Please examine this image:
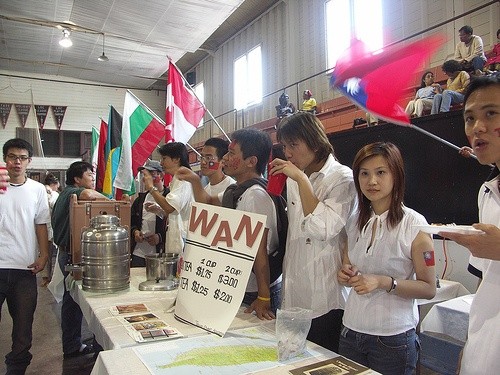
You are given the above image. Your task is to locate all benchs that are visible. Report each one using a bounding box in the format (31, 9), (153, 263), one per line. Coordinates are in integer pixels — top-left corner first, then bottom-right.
(188, 65), (463, 164)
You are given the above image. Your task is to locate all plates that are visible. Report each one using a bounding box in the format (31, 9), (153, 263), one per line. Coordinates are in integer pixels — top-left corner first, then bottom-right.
(411, 225), (485, 235)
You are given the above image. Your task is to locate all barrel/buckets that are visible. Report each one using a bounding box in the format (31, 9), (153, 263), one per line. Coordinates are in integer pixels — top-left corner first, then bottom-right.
(81, 215), (131, 293)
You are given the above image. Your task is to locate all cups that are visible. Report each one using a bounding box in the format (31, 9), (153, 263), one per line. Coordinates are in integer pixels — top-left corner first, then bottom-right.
(267, 163), (288, 196)
(0, 162), (7, 191)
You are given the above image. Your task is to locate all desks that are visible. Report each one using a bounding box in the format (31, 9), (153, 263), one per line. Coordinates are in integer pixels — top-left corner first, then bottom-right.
(416, 279), (472, 375)
(91, 323), (383, 375)
(420, 294), (475, 342)
(47, 248), (276, 349)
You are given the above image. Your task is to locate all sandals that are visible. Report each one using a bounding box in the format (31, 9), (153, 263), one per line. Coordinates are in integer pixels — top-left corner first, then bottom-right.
(64, 344), (94, 357)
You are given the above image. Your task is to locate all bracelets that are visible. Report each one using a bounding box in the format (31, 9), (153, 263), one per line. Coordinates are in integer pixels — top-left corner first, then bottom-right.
(257, 296), (270, 301)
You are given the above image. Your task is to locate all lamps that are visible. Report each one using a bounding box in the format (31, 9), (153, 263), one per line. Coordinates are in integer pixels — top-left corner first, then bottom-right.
(59, 27), (73, 47)
(98, 32), (109, 62)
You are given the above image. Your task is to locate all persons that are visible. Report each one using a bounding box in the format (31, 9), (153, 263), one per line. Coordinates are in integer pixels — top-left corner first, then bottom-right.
(0, 168), (10, 194)
(275, 92), (296, 124)
(336, 141), (437, 375)
(0, 26), (500, 375)
(268, 112), (355, 357)
(142, 142), (194, 261)
(51, 161), (111, 356)
(0, 138), (51, 375)
(201, 137), (237, 195)
(131, 158), (170, 267)
(224, 129), (279, 320)
(296, 90), (316, 115)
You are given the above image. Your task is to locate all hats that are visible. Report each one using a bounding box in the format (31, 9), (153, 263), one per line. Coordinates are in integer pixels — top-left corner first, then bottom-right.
(137, 160), (163, 172)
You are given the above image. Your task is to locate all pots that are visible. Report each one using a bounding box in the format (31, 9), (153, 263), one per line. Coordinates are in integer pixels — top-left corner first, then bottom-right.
(144, 252), (179, 281)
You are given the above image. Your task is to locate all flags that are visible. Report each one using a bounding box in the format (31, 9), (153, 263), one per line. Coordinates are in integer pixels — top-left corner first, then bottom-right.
(97, 120), (107, 194)
(162, 62), (207, 144)
(112, 92), (167, 192)
(91, 127), (100, 189)
(103, 108), (135, 200)
(330, 33), (445, 125)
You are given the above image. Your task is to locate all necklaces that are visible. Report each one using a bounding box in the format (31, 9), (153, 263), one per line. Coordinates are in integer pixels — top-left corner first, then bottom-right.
(10, 176), (27, 187)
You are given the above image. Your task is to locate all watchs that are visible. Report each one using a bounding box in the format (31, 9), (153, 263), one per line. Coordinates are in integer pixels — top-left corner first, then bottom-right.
(386, 277), (397, 293)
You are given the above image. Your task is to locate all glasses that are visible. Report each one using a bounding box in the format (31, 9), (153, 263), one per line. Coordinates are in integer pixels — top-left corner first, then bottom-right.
(5, 153), (30, 161)
(197, 156), (222, 161)
